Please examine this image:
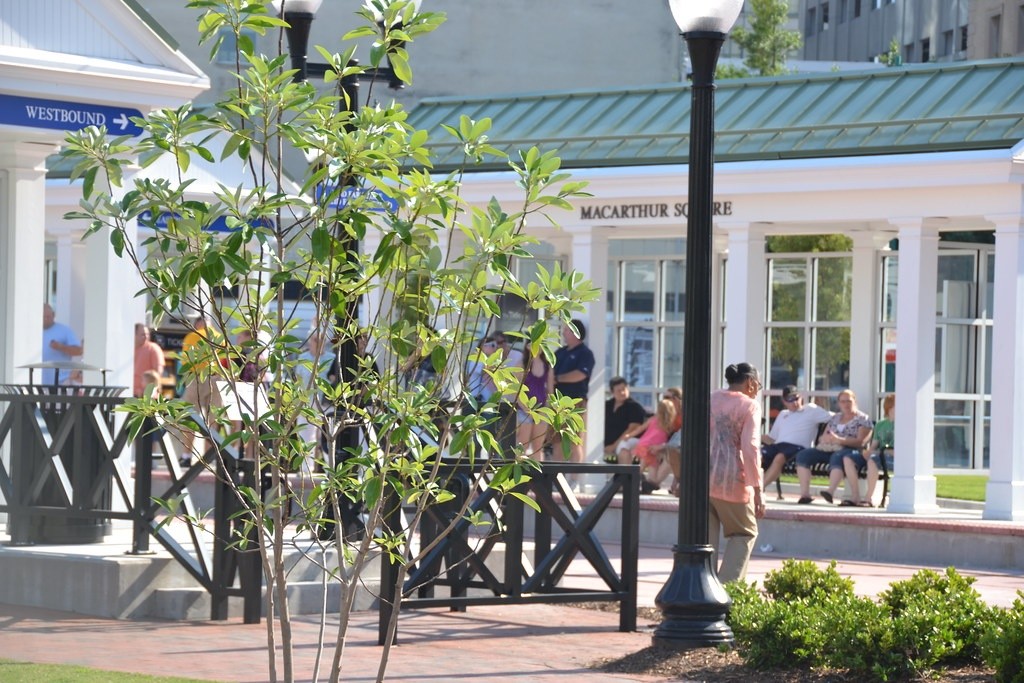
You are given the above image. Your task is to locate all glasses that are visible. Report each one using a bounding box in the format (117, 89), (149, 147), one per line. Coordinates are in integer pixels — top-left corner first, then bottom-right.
(750, 375), (763, 390)
(784, 396), (800, 402)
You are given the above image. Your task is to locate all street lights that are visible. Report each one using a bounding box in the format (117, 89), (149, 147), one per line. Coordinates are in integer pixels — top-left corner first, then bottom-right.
(650, 0), (744, 660)
(278, 2), (425, 547)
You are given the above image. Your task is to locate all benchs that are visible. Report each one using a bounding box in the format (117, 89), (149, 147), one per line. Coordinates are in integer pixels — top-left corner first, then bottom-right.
(766, 415), (895, 508)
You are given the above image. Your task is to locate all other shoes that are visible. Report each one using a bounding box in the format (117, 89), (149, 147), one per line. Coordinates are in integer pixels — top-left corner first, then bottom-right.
(798, 497), (814, 504)
(820, 491), (834, 503)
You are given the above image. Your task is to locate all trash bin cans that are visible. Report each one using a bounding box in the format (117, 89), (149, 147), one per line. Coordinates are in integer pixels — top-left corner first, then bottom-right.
(2, 356), (131, 539)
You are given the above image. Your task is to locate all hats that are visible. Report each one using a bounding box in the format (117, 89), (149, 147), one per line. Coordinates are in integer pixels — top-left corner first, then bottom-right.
(782, 384), (798, 398)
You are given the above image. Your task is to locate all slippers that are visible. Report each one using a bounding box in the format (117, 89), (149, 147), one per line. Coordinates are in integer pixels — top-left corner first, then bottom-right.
(857, 501), (874, 507)
(838, 500), (857, 507)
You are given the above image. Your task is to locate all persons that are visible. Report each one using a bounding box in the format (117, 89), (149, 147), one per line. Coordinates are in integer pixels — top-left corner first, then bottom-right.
(707, 362), (768, 585)
(760, 384), (841, 495)
(839, 395), (895, 507)
(173, 287), (680, 544)
(41, 303), (85, 385)
(134, 324), (166, 467)
(797, 390), (875, 503)
